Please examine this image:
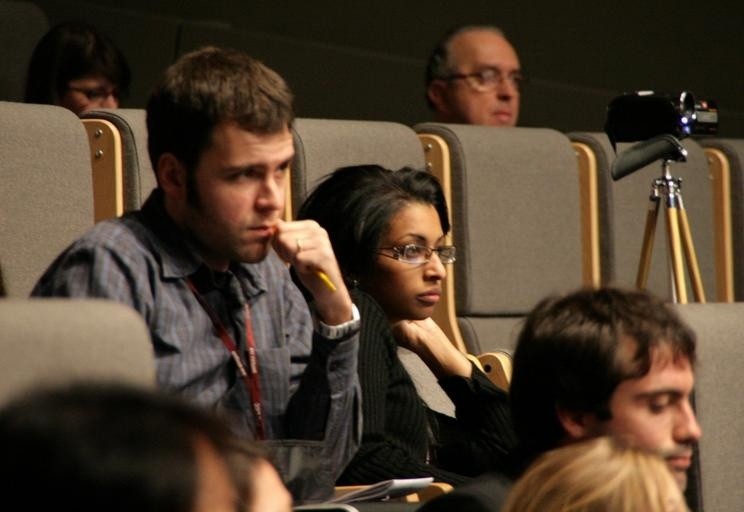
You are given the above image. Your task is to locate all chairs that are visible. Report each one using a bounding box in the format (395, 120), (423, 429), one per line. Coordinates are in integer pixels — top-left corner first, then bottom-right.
(2, 92), (95, 308)
(650, 306), (740, 507)
(275, 112), (450, 334)
(423, 114), (581, 308)
(97, 98), (262, 298)
(581, 118), (735, 303)
(0, 287), (164, 510)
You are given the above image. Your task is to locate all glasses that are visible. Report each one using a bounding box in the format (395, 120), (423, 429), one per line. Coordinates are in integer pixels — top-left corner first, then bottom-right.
(451, 70), (523, 89)
(372, 245), (457, 268)
(67, 83), (131, 100)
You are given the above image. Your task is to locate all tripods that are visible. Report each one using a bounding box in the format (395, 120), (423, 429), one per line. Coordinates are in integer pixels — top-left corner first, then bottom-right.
(635, 176), (707, 304)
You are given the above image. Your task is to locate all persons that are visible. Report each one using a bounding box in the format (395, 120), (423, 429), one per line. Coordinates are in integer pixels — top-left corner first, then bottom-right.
(29, 24), (121, 113)
(290, 163), (512, 493)
(415, 285), (704, 512)
(498, 434), (689, 512)
(218, 424), (295, 512)
(0, 379), (242, 512)
(26, 44), (365, 510)
(423, 22), (526, 127)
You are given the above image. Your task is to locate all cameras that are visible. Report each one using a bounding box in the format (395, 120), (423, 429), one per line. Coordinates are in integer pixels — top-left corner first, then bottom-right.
(603, 91), (720, 146)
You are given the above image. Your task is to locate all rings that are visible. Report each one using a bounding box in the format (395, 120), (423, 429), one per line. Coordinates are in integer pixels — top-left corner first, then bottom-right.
(295, 240), (305, 252)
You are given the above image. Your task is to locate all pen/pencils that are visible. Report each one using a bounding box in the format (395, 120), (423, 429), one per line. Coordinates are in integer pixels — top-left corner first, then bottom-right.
(315, 270), (336, 292)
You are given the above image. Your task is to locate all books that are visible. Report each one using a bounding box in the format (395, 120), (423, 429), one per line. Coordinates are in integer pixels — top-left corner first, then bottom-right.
(321, 476), (435, 504)
